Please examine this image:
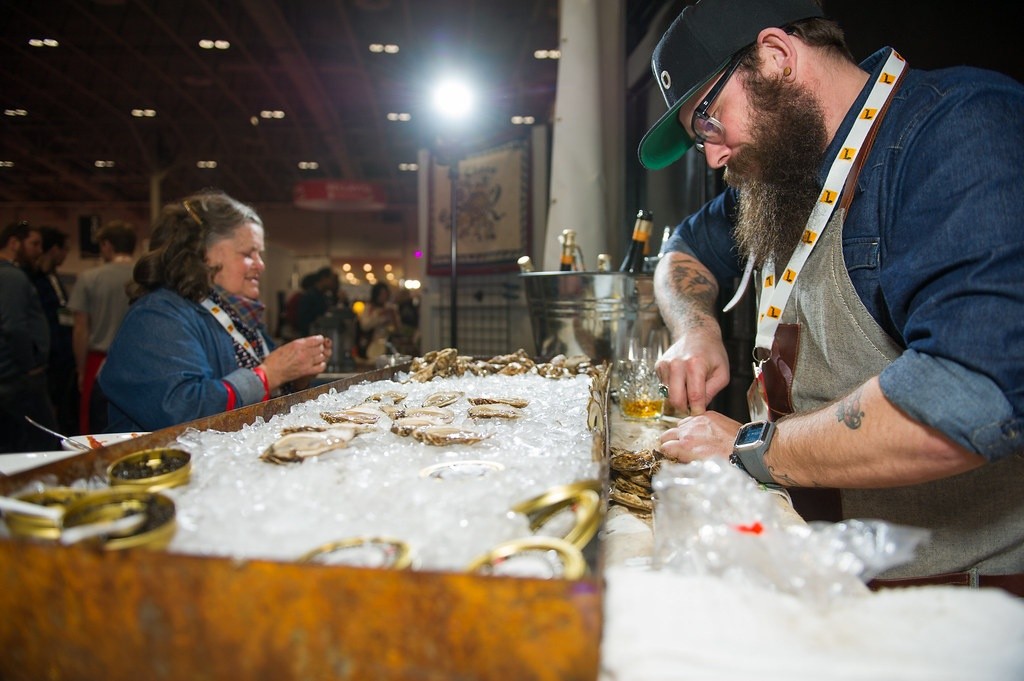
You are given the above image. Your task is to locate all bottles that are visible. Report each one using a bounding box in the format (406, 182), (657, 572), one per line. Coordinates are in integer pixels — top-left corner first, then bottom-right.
(597, 254), (612, 271)
(619, 209), (653, 273)
(517, 255), (536, 273)
(559, 230), (586, 271)
(660, 226), (674, 249)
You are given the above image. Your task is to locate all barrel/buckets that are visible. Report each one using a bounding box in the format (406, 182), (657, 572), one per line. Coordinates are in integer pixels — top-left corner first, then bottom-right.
(519, 271), (671, 359)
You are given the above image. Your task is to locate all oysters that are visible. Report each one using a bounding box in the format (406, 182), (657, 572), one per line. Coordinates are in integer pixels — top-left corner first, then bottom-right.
(254, 347), (612, 468)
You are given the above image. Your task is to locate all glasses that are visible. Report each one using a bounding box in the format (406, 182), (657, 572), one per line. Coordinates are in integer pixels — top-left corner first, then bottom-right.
(17, 220), (28, 226)
(691, 27), (795, 155)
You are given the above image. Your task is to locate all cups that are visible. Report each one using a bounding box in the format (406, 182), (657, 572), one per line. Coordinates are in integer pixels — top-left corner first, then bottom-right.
(622, 347), (666, 421)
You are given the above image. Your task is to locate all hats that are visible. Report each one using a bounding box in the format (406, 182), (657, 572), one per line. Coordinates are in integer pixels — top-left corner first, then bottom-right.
(637, 0), (825, 171)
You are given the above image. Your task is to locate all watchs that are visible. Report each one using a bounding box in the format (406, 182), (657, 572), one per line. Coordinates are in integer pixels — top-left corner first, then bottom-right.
(732, 420), (785, 490)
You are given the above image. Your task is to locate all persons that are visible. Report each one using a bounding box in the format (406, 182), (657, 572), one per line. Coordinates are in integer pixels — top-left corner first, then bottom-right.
(0, 219), (139, 454)
(638, 0), (1023, 606)
(276, 267), (420, 372)
(86, 191), (335, 439)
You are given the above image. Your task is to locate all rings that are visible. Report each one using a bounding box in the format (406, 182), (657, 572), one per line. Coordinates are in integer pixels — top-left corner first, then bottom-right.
(657, 383), (670, 398)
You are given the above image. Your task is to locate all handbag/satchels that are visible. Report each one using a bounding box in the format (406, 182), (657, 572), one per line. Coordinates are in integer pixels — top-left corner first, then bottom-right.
(354, 322), (375, 358)
